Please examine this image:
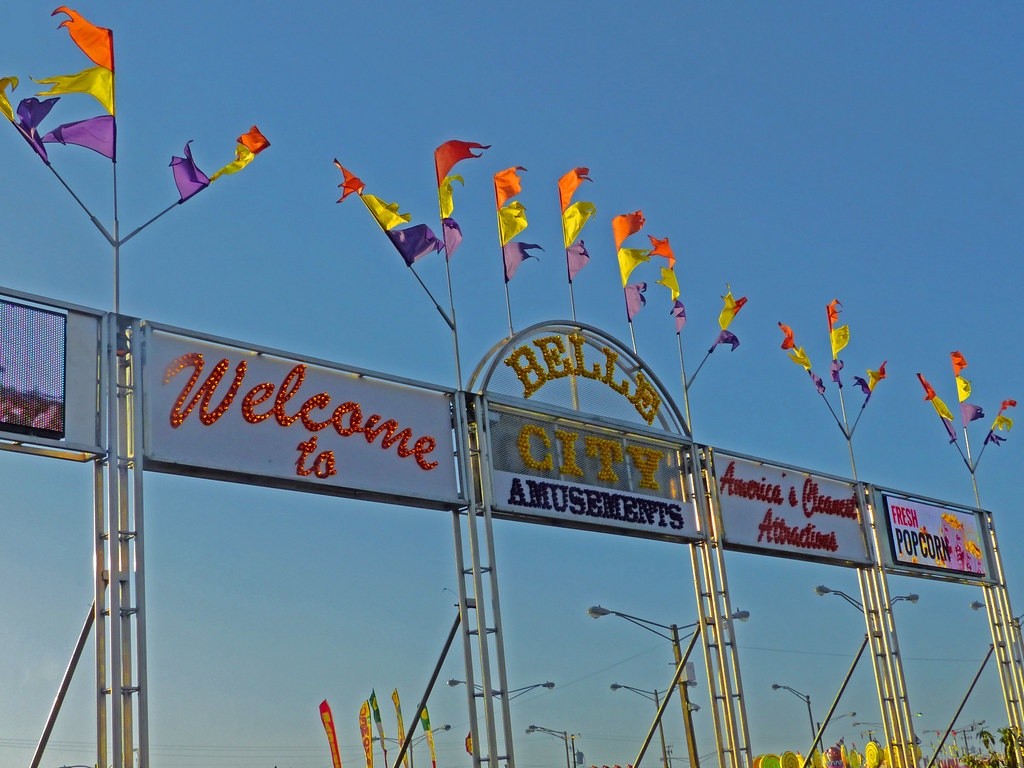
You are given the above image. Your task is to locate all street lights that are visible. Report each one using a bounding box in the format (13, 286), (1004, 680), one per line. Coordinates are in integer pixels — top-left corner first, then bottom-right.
(587, 604), (750, 768)
(962, 721), (987, 754)
(526, 724), (570, 768)
(370, 725), (453, 768)
(816, 712), (858, 754)
(922, 728), (960, 758)
(609, 680), (700, 768)
(772, 683), (816, 743)
(970, 600), (1024, 668)
